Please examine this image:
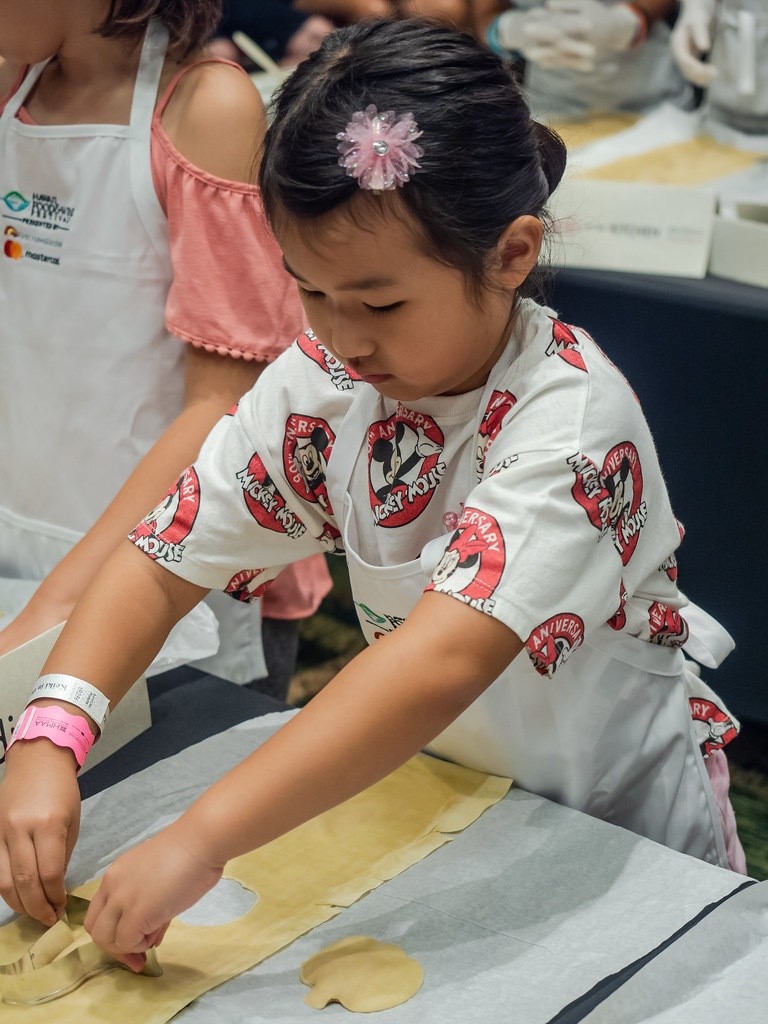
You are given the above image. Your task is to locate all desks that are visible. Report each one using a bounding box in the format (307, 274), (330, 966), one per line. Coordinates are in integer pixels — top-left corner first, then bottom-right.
(525, 97), (768, 769)
(1, 662), (768, 1024)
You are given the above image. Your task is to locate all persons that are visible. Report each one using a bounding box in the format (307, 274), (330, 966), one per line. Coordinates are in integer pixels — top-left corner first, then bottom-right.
(199, 0), (670, 68)
(0, 1), (335, 697)
(0, 1), (750, 976)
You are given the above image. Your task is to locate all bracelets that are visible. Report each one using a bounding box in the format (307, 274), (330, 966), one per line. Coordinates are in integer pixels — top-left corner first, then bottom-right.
(3, 671), (113, 776)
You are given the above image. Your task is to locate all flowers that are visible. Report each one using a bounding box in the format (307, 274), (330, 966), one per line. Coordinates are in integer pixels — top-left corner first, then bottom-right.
(335, 104), (425, 196)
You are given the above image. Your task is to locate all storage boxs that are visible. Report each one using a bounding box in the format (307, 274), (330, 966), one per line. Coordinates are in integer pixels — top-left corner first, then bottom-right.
(1, 574), (151, 780)
